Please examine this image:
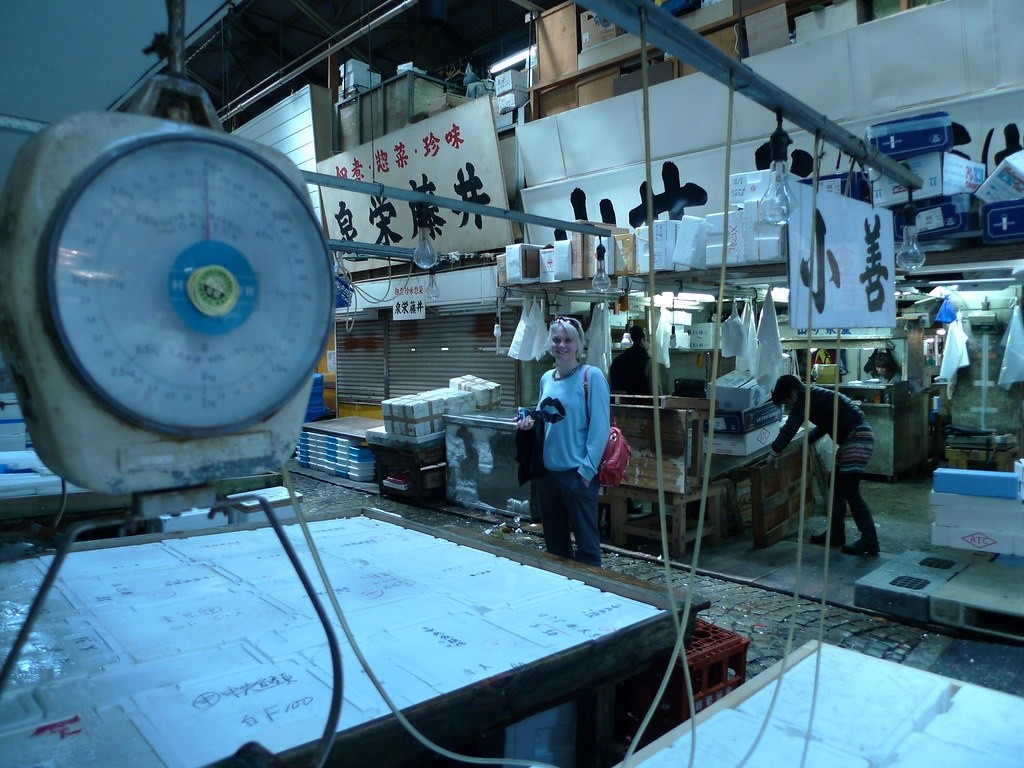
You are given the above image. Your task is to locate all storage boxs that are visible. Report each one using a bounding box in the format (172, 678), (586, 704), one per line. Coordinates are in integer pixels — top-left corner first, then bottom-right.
(928, 458), (1024, 568)
(292, 372), (502, 509)
(813, 363), (837, 383)
(671, 368), (795, 524)
(669, 616), (752, 727)
(339, 1), (1024, 290)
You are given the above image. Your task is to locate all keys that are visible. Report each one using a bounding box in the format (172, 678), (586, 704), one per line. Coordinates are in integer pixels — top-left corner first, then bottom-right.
(529, 418), (535, 422)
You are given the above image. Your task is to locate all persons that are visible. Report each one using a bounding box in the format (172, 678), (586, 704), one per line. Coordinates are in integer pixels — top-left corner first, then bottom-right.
(515, 316), (632, 567)
(767, 374), (881, 555)
(864, 355), (901, 382)
(609, 325), (663, 406)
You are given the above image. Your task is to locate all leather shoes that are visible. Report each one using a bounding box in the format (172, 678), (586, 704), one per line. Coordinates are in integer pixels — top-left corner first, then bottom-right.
(842, 539), (880, 553)
(810, 531), (845, 544)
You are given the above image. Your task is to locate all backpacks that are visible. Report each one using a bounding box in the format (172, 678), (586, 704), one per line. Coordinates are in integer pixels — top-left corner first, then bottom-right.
(597, 407), (632, 487)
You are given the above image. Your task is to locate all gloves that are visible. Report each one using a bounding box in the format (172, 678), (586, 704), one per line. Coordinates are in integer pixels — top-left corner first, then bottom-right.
(767, 454), (782, 469)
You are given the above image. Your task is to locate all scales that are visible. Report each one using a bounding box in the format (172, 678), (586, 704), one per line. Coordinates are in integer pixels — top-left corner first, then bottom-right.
(0, 0), (340, 768)
